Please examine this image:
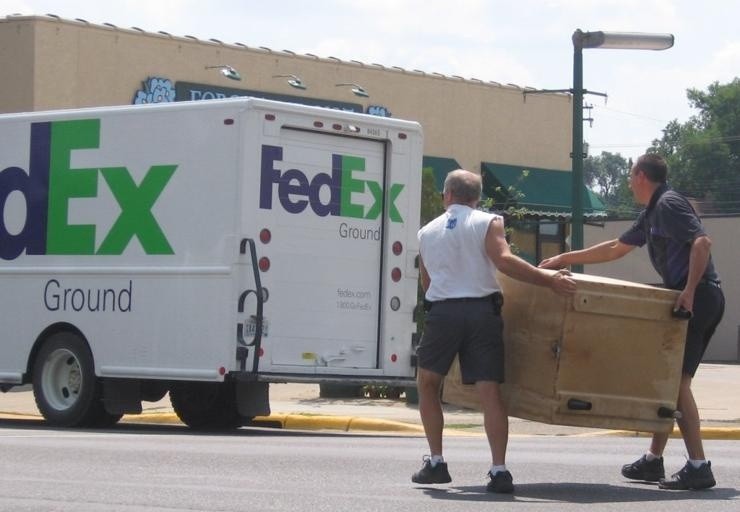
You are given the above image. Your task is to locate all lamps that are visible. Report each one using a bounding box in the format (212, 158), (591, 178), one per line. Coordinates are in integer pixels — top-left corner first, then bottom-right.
(205, 63), (371, 99)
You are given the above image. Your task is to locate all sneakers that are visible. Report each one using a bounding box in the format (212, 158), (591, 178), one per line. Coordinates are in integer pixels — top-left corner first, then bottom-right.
(486, 470), (514, 493)
(623, 455), (664, 481)
(658, 461), (716, 489)
(412, 459), (451, 484)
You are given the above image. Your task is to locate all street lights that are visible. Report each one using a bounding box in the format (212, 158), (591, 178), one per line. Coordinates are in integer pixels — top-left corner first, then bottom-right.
(569, 27), (675, 276)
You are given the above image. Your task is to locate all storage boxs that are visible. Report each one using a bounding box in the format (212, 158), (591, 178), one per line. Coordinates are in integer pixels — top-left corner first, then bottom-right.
(440, 266), (692, 434)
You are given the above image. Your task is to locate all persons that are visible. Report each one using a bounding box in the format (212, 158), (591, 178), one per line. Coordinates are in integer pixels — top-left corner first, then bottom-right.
(535, 152), (725, 490)
(411, 166), (579, 493)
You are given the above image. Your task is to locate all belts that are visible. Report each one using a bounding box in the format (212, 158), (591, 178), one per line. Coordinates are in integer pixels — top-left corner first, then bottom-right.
(702, 278), (720, 288)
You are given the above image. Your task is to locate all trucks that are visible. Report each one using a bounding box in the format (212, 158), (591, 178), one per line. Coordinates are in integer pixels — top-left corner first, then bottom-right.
(0, 95), (428, 434)
